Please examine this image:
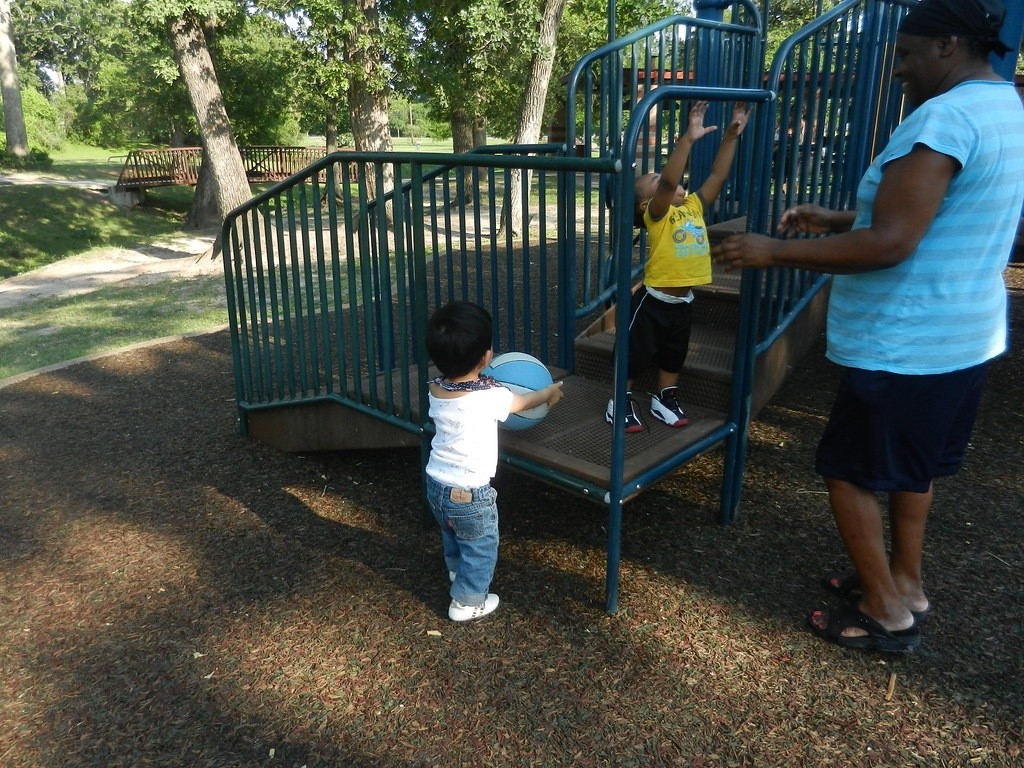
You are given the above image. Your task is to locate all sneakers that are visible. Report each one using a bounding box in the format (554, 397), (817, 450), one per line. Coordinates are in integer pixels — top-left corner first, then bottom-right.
(448, 592), (499, 622)
(650, 384), (689, 426)
(449, 571), (456, 583)
(605, 391), (644, 433)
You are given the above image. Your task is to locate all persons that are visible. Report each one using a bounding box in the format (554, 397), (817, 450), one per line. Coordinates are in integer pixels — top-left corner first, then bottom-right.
(426, 303), (564, 622)
(711, 0), (1024, 654)
(603, 99), (752, 433)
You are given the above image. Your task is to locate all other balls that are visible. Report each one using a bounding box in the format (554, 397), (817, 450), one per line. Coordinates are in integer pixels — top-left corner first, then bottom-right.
(482, 352), (554, 431)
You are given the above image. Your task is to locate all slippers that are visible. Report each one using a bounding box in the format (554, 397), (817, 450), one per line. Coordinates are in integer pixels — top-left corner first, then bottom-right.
(826, 574), (931, 624)
(807, 603), (922, 652)
